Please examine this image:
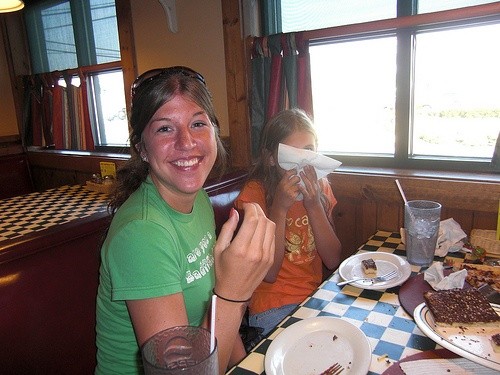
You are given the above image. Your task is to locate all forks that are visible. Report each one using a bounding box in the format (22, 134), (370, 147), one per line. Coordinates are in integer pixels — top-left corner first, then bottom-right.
(336, 270), (399, 286)
(320, 362), (344, 375)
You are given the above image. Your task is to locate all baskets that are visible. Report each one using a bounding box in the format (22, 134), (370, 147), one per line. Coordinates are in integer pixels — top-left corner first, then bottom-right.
(470, 229), (500, 254)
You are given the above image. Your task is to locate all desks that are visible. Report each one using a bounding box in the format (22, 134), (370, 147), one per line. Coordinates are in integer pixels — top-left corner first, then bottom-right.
(0, 182), (122, 243)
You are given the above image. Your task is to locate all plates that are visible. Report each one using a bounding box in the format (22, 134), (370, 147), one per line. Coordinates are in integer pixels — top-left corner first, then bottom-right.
(338, 252), (412, 290)
(413, 302), (500, 372)
(265, 316), (372, 375)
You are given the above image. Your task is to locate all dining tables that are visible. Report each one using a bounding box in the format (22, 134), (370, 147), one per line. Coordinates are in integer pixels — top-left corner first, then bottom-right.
(226, 230), (500, 375)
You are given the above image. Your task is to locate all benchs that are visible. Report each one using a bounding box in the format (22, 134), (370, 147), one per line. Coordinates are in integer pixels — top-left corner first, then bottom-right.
(0, 153), (32, 200)
(0, 165), (259, 375)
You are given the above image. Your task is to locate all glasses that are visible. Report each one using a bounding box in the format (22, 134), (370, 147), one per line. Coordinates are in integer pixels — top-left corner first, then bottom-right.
(130, 66), (205, 105)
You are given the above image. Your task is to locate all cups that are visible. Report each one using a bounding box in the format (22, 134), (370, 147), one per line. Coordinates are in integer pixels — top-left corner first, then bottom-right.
(404, 200), (442, 266)
(142, 325), (219, 375)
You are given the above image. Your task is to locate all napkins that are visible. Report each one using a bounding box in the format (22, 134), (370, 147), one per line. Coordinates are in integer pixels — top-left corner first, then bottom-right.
(400, 217), (467, 257)
(278, 143), (342, 187)
(424, 261), (467, 291)
(399, 358), (500, 375)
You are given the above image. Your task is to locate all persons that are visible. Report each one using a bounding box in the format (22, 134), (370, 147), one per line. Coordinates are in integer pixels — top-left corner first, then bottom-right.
(236, 110), (342, 338)
(95, 68), (276, 375)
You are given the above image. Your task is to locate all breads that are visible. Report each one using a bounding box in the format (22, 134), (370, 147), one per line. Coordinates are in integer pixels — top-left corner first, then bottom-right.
(362, 259), (377, 274)
(452, 262), (500, 292)
(424, 289), (500, 350)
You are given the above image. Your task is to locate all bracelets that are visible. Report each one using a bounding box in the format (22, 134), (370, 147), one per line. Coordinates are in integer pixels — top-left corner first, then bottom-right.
(212, 288), (251, 302)
